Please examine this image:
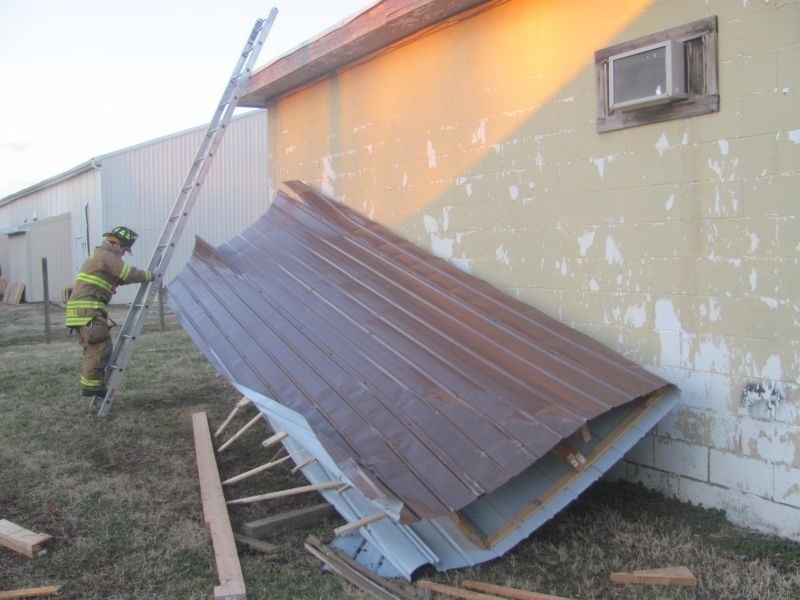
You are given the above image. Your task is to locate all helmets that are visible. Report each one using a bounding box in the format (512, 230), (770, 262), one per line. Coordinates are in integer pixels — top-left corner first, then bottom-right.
(102, 227), (139, 246)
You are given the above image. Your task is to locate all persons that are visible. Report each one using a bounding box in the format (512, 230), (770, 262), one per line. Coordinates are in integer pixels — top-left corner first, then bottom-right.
(68, 226), (155, 398)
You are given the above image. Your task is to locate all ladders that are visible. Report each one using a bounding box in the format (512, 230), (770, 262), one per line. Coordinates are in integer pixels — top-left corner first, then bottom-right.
(90, 6), (279, 418)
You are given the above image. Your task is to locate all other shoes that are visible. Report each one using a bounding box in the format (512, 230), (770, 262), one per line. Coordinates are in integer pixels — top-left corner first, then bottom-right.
(82, 387), (114, 403)
